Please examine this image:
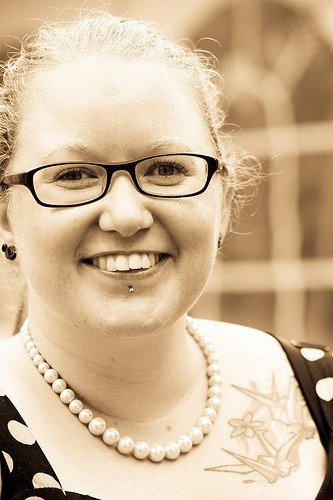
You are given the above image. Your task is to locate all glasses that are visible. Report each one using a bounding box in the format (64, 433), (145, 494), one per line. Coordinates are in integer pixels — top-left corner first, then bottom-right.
(1, 153), (224, 207)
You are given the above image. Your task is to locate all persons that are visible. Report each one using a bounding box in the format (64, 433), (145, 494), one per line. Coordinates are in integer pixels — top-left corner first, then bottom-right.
(0, 13), (332, 500)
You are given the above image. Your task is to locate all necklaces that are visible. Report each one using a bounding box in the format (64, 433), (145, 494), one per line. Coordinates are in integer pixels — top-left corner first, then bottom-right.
(19, 315), (224, 463)
